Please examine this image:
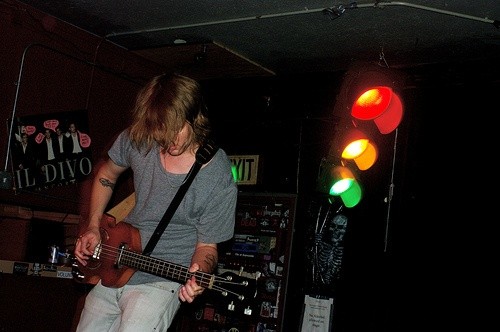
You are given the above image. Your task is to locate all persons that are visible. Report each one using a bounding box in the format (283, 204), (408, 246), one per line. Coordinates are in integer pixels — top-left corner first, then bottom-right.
(58, 69), (243, 332)
(9, 117), (84, 170)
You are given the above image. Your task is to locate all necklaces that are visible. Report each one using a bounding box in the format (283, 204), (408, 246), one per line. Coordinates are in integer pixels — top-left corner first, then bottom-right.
(161, 152), (200, 188)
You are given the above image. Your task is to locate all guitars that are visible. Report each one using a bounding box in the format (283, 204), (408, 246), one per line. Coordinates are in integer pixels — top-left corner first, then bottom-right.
(72, 212), (261, 315)
